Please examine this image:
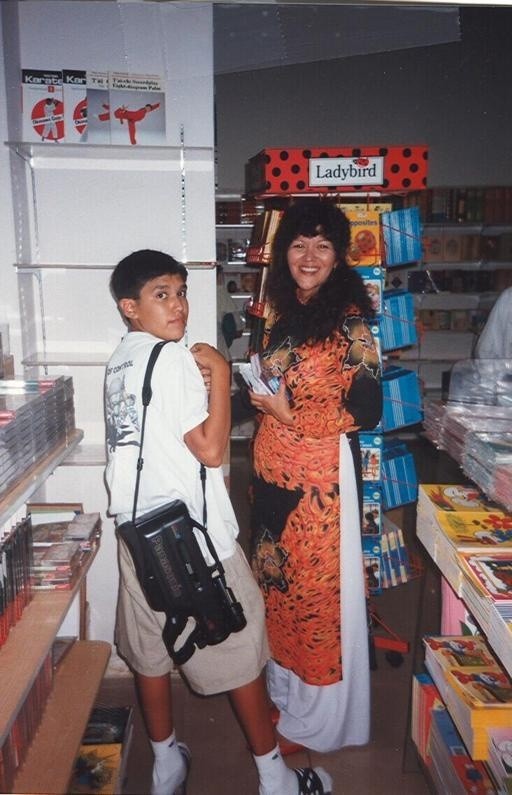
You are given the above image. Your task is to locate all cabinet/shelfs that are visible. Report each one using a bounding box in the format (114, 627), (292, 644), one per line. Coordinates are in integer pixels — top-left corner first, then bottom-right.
(3, 125), (215, 477)
(216, 180), (510, 441)
(0, 416), (142, 794)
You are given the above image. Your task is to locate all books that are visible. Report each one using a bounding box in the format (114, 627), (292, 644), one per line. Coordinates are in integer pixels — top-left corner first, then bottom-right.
(0, 372), (102, 794)
(403, 187), (512, 332)
(240, 351), (292, 413)
(81, 703), (132, 742)
(409, 390), (512, 794)
(68, 743), (123, 795)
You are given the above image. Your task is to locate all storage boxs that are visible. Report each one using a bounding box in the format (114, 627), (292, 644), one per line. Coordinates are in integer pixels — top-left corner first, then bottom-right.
(243, 142), (432, 195)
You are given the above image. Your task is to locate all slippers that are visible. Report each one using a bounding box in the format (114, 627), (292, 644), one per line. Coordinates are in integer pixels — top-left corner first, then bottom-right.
(258, 766), (333, 795)
(150, 742), (193, 795)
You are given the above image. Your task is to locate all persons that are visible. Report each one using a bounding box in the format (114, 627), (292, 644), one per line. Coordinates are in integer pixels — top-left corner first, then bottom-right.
(100, 244), (333, 795)
(357, 448), (383, 594)
(232, 198), (383, 760)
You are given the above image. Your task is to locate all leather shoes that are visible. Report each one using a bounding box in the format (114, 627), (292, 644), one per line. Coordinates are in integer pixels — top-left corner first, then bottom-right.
(277, 734), (304, 755)
(268, 703), (281, 725)
